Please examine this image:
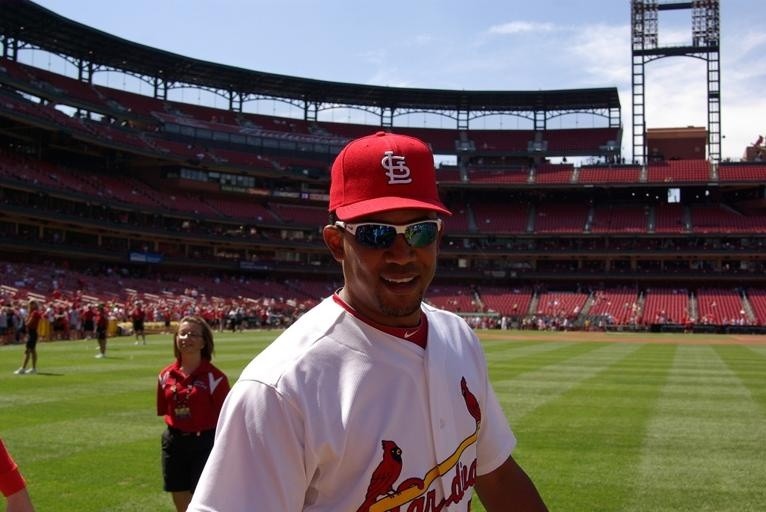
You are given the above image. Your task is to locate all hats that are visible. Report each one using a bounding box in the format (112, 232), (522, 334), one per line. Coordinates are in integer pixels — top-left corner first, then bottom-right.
(329, 131), (452, 220)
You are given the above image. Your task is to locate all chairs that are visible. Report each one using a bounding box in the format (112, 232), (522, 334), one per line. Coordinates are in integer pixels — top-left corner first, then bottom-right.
(0, 55), (766, 339)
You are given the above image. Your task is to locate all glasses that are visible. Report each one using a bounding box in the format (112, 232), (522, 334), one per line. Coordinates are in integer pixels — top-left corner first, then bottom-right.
(335, 219), (441, 250)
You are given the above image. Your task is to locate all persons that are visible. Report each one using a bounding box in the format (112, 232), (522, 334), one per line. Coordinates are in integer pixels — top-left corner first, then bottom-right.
(182, 130), (554, 512)
(0, 439), (33, 512)
(2, 237), (760, 341)
(91, 304), (109, 359)
(155, 314), (231, 512)
(13, 300), (40, 375)
(129, 302), (147, 345)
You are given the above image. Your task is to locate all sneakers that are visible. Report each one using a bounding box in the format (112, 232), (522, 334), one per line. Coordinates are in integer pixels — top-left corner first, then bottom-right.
(96, 353), (107, 359)
(24, 368), (37, 374)
(14, 367), (24, 374)
